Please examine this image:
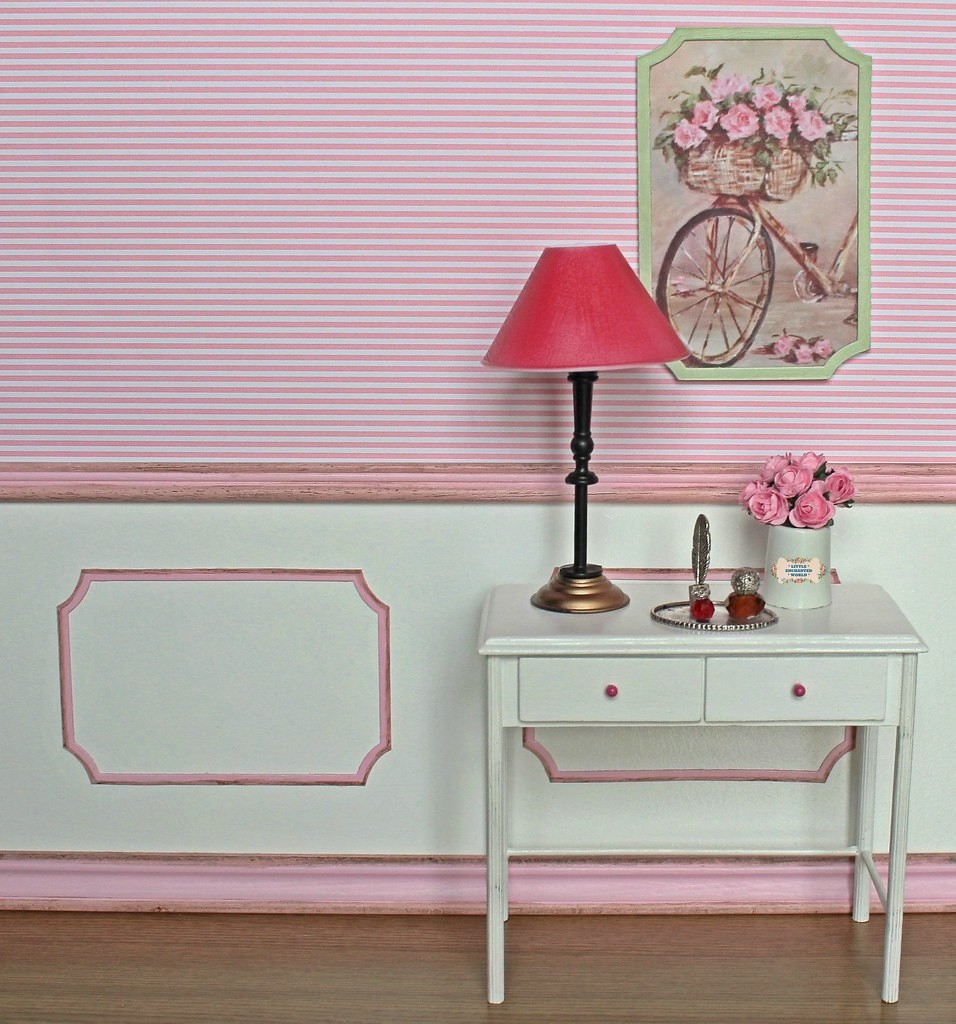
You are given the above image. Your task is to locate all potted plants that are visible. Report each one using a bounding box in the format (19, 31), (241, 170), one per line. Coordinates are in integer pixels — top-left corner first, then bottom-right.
(740, 451), (857, 617)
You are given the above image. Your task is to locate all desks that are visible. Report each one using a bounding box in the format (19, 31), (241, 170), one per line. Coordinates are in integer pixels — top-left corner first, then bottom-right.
(474, 575), (932, 1008)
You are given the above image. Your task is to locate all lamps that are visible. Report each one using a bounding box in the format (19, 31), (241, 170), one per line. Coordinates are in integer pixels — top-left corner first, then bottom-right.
(480, 241), (692, 618)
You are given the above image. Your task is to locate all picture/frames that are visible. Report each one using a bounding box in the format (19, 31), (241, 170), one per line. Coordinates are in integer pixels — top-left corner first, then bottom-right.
(633, 22), (877, 385)
